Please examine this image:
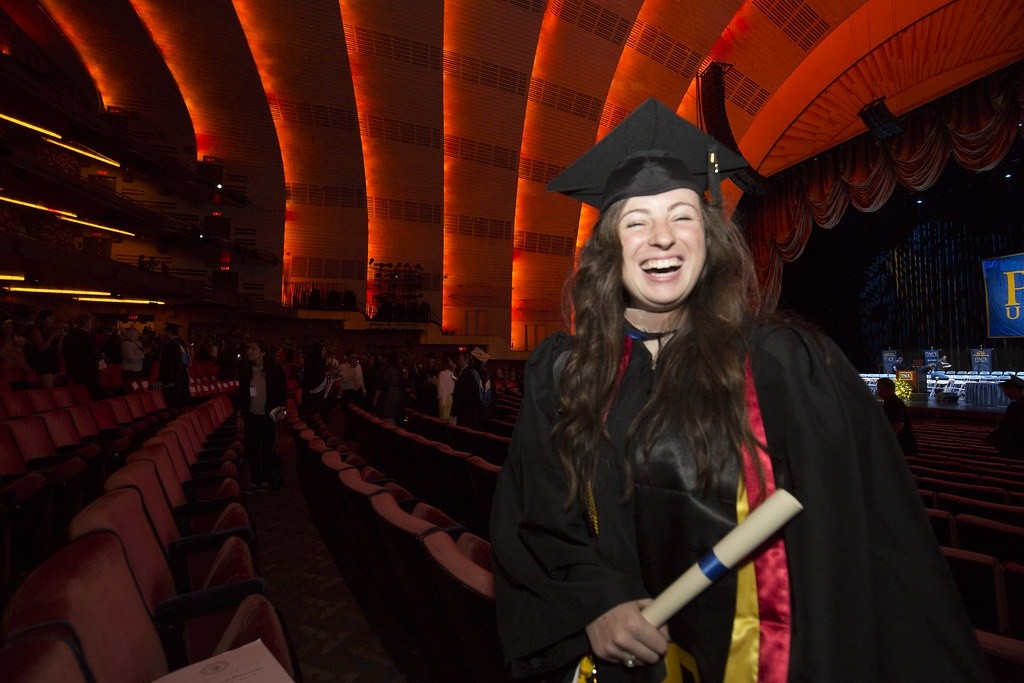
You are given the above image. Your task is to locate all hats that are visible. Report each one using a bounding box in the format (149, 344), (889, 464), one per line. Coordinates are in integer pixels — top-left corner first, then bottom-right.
(546, 98), (747, 224)
(164, 322), (181, 330)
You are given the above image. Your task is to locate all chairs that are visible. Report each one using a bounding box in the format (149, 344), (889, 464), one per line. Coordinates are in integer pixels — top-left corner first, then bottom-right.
(0, 316), (1024, 683)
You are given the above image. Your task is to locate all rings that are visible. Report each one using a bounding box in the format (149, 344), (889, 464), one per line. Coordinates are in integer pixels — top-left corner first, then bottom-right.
(624, 655), (635, 667)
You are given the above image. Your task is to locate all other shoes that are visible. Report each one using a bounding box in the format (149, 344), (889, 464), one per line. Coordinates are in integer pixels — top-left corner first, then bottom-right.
(244, 483), (257, 495)
(258, 481), (270, 492)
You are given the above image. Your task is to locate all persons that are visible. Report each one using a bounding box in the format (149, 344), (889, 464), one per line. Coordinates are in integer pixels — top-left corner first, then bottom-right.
(490, 98), (997, 682)
(0, 304), (193, 408)
(198, 334), (282, 362)
(286, 345), (296, 361)
(236, 340), (289, 494)
(367, 346), (492, 426)
(391, 283), (431, 323)
(300, 342), (366, 410)
(984, 375), (1024, 459)
(877, 379), (919, 456)
